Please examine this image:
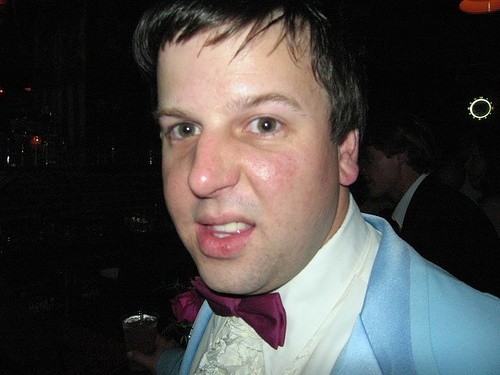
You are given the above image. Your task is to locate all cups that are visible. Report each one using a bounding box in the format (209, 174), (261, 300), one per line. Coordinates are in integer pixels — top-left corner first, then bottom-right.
(122, 314), (159, 371)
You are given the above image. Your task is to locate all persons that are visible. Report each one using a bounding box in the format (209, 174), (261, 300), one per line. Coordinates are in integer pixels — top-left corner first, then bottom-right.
(122, 0), (500, 375)
(358, 112), (500, 298)
(427, 113), (500, 232)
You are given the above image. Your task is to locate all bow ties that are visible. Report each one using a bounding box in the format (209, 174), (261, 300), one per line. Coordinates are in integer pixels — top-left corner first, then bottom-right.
(191, 276), (286, 351)
